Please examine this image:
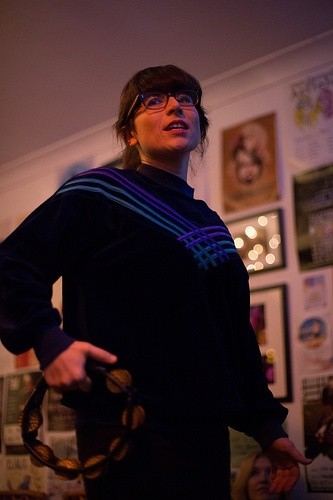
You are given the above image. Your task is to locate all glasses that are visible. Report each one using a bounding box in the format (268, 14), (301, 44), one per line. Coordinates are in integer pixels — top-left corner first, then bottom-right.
(124, 91), (199, 123)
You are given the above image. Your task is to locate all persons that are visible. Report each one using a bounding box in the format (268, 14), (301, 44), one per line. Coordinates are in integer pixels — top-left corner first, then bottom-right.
(231, 450), (284, 500)
(1, 62), (313, 500)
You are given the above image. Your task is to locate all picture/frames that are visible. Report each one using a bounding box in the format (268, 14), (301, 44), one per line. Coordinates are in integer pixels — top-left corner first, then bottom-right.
(249, 282), (294, 403)
(223, 207), (287, 275)
(219, 110), (282, 216)
